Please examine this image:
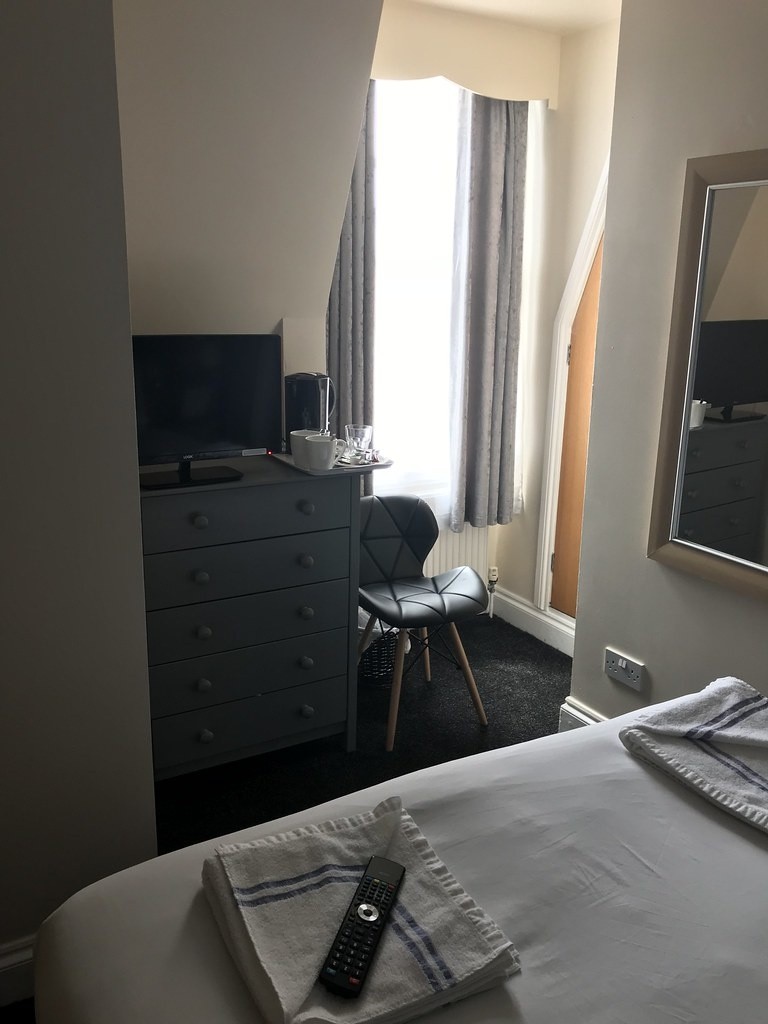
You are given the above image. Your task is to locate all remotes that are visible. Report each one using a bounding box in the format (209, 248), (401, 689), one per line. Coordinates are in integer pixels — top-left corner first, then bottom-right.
(318, 855), (406, 1000)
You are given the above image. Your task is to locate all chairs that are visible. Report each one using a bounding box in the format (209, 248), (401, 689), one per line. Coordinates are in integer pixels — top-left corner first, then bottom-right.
(353, 495), (488, 751)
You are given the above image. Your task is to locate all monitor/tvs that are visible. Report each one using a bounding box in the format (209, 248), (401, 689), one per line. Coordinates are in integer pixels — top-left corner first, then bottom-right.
(693, 319), (768, 423)
(133, 334), (283, 491)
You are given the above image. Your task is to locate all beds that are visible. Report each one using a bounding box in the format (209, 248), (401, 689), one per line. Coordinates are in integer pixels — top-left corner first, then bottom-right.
(23, 674), (768, 1024)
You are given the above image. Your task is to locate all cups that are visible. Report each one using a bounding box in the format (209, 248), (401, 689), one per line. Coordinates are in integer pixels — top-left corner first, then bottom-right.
(345, 424), (373, 459)
(304, 429), (330, 436)
(290, 430), (324, 466)
(305, 436), (346, 471)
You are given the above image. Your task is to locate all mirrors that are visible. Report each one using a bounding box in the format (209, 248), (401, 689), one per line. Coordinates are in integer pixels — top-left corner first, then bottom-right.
(644, 148), (768, 602)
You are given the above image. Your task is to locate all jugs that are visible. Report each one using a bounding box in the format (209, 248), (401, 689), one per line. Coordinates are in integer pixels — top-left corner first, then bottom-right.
(285, 372), (336, 455)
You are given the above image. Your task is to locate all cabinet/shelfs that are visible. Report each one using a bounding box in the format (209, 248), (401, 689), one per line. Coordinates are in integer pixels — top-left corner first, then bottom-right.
(678, 416), (768, 562)
(140, 454), (372, 781)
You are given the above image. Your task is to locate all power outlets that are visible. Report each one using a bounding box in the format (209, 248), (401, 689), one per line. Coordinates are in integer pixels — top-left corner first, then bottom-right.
(603, 644), (646, 693)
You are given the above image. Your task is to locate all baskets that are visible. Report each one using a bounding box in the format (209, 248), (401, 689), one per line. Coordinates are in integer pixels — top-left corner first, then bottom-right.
(359, 628), (398, 687)
